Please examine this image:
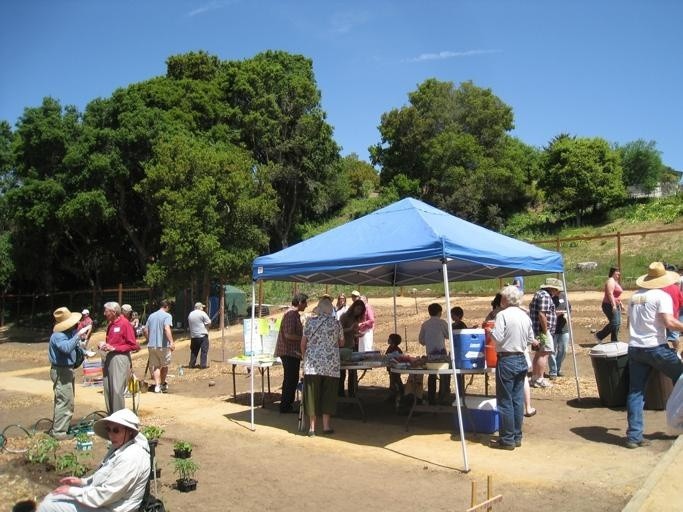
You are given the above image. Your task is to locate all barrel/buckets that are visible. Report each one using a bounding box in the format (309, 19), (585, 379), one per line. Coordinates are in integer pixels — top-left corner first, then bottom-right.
(482, 320), (498, 367)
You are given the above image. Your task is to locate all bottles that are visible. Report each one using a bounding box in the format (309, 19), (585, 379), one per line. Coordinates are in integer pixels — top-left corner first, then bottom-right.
(177, 364), (183, 376)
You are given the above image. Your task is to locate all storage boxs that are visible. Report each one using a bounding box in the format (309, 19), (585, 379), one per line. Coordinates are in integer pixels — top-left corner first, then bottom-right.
(452, 395), (500, 434)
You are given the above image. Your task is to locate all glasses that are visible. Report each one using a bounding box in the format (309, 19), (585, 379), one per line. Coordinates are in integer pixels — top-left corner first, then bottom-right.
(105, 427), (119, 433)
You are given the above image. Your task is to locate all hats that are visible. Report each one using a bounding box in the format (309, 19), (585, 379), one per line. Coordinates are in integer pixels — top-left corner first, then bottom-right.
(539, 278), (564, 292)
(635, 261), (679, 289)
(194, 302), (205, 307)
(350, 290), (359, 296)
(93, 408), (139, 440)
(52, 307), (82, 332)
(318, 294), (333, 302)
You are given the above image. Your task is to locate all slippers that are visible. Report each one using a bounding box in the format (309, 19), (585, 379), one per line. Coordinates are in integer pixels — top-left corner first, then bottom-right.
(526, 408), (535, 417)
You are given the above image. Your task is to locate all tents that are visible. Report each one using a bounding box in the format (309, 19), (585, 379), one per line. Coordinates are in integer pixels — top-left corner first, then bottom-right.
(176, 284), (248, 329)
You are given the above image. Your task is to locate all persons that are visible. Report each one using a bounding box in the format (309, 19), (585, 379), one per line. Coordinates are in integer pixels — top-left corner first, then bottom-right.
(187, 302), (211, 369)
(272, 290), (374, 436)
(76, 302), (140, 416)
(385, 333), (404, 394)
(38, 408), (151, 512)
(143, 300), (175, 394)
(48, 306), (96, 436)
(486, 261), (683, 451)
(451, 307), (467, 398)
(419, 303), (449, 406)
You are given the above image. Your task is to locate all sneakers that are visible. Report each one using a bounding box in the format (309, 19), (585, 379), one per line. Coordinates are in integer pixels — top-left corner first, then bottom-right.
(53, 435), (73, 441)
(488, 439), (520, 450)
(626, 439), (651, 448)
(530, 372), (564, 388)
(154, 380), (167, 392)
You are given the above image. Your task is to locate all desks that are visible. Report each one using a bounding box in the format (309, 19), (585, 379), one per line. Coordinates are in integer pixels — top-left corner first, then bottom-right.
(337, 360), (387, 422)
(390, 362), (500, 434)
(227, 356), (299, 409)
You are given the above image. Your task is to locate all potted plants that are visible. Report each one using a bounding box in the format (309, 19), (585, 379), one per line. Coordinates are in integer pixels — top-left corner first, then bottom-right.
(173, 440), (193, 459)
(141, 425), (166, 457)
(150, 462), (161, 479)
(173, 459), (199, 491)
(74, 433), (94, 452)
